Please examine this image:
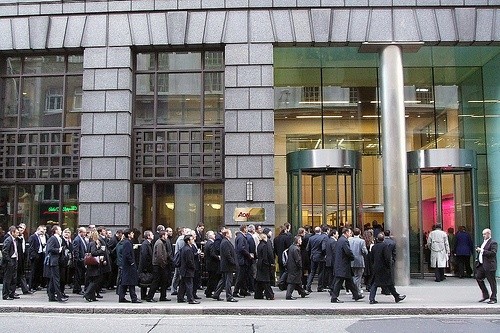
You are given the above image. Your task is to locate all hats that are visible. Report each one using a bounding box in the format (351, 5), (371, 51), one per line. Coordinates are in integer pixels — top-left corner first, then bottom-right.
(263, 228), (271, 234)
(436, 223), (442, 227)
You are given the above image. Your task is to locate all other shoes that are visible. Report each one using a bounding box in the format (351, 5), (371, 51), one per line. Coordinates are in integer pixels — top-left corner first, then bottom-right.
(435, 279), (440, 282)
(381, 291), (391, 295)
(395, 295), (406, 303)
(479, 298), (487, 302)
(368, 288), (371, 291)
(459, 275), (464, 278)
(370, 300), (378, 304)
(467, 274), (471, 278)
(488, 299), (496, 303)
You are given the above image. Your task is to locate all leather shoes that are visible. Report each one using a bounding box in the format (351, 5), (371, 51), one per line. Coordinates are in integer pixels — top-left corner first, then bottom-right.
(2, 285), (366, 304)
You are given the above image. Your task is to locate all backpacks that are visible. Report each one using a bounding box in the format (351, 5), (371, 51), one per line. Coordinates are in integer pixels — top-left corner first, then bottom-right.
(281, 248), (288, 266)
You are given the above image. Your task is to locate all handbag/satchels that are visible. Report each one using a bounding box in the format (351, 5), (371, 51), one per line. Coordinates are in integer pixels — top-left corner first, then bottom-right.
(85, 253), (100, 266)
(171, 248), (181, 268)
(139, 271), (153, 287)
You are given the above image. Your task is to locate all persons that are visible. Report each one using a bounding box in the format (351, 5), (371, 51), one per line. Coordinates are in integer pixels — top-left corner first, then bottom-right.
(426, 223), (450, 282)
(330, 228), (365, 303)
(171, 222), (205, 298)
(2, 223), (33, 300)
(456, 226), (473, 277)
(212, 229), (239, 302)
(369, 233), (406, 304)
(214, 225), (228, 292)
(274, 221), (352, 300)
(112, 228), (142, 303)
(177, 236), (200, 304)
(235, 223), (276, 299)
(72, 224), (111, 302)
(204, 231), (221, 298)
(348, 219), (396, 298)
(140, 224), (173, 302)
(410, 226), (428, 255)
(474, 228), (498, 303)
(447, 227), (458, 274)
(29, 224), (73, 302)
